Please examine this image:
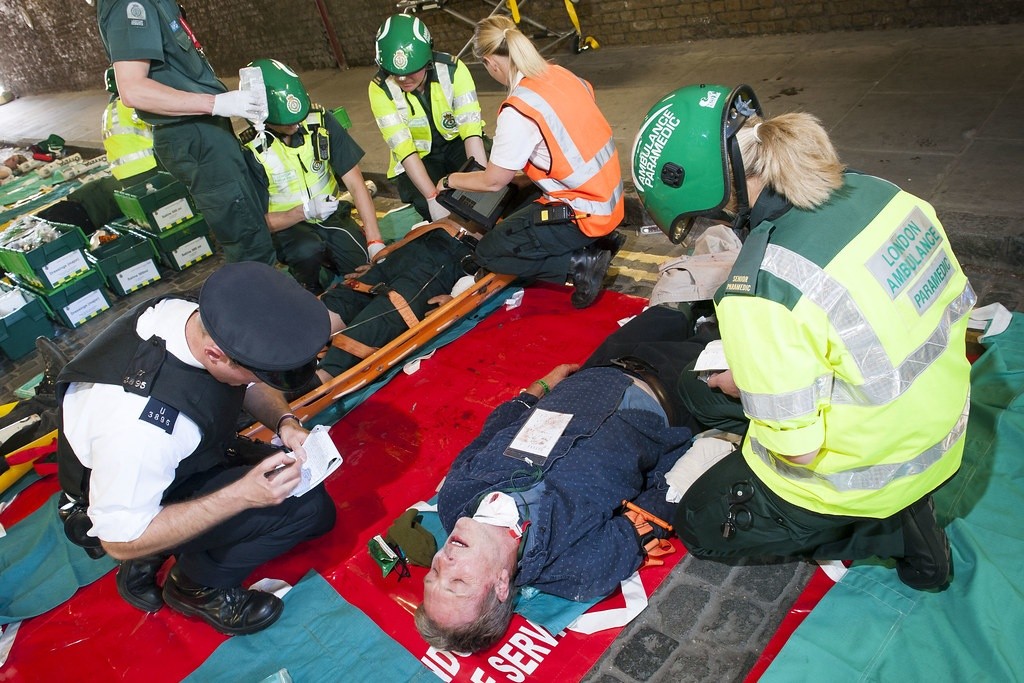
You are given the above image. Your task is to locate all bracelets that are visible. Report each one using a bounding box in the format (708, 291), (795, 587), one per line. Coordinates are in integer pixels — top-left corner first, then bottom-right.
(443, 173), (451, 188)
(276, 413), (303, 438)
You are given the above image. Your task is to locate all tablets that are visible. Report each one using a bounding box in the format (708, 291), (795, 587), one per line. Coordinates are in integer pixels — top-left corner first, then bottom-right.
(443, 162), (517, 230)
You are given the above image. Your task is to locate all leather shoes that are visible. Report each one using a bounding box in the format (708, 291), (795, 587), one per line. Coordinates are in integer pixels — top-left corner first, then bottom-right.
(594, 231), (627, 261)
(162, 573), (283, 636)
(35, 336), (71, 393)
(117, 557), (161, 613)
(571, 246), (611, 309)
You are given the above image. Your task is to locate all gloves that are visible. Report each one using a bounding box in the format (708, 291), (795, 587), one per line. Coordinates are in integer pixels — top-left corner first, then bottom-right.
(427, 195), (451, 221)
(212, 90), (263, 119)
(367, 242), (387, 264)
(304, 195), (339, 221)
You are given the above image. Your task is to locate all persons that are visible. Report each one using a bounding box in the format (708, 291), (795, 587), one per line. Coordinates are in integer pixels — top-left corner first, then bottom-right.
(630, 82), (977, 594)
(413, 293), (720, 655)
(100, 67), (160, 187)
(284, 213), (489, 403)
(97, 0), (273, 266)
(239, 58), (386, 295)
(55, 260), (336, 634)
(436, 15), (627, 308)
(368, 14), (490, 224)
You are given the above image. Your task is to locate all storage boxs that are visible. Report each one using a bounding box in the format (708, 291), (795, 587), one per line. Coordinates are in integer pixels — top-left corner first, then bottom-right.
(4, 258), (113, 332)
(0, 281), (55, 362)
(0, 214), (93, 292)
(83, 225), (162, 298)
(112, 212), (216, 273)
(112, 171), (198, 235)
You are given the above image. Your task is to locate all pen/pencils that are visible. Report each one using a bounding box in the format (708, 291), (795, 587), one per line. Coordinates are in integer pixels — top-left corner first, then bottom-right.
(326, 195), (329, 203)
(263, 463), (293, 478)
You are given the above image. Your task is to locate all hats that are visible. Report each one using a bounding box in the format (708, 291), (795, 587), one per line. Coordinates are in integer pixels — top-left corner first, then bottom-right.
(199, 261), (331, 392)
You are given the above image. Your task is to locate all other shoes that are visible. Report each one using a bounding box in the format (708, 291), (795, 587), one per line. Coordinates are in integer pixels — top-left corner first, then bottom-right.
(897, 496), (950, 591)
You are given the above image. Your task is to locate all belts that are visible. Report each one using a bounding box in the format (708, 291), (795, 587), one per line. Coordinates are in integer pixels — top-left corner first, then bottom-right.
(152, 116), (200, 132)
(612, 357), (681, 428)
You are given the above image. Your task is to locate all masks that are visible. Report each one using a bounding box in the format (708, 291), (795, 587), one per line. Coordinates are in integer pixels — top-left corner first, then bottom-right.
(472, 491), (520, 526)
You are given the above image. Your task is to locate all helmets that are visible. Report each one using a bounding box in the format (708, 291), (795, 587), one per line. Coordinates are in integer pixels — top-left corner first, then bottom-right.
(103, 67), (119, 93)
(246, 58), (311, 125)
(375, 14), (434, 75)
(630, 84), (763, 245)
